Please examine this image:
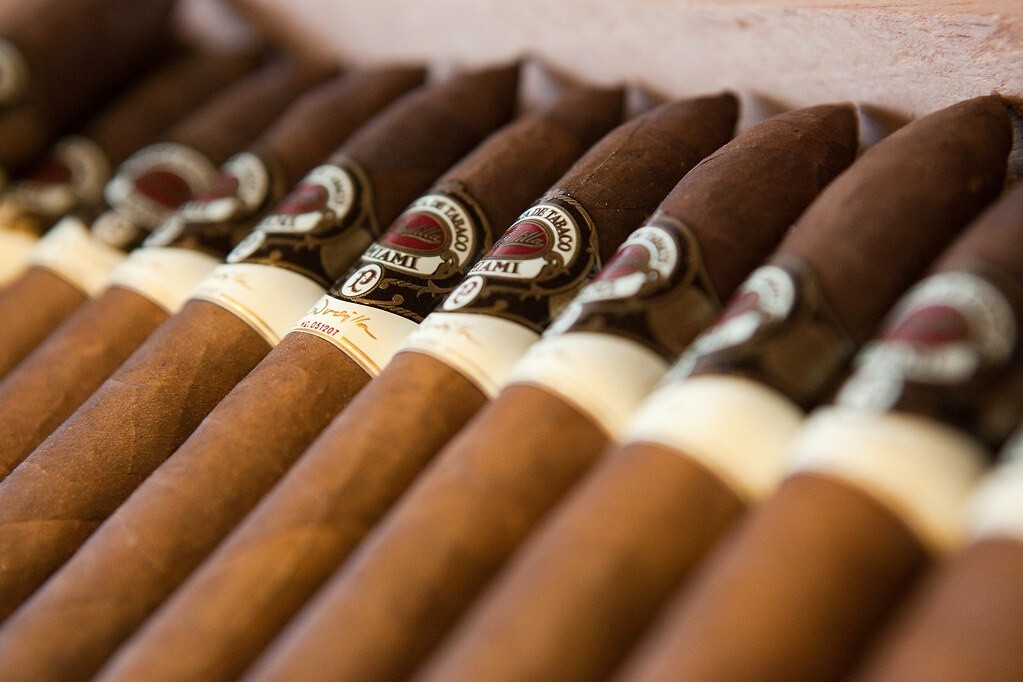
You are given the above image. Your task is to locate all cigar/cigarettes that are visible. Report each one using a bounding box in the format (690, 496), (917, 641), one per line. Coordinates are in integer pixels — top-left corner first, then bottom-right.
(0, 30), (1023, 682)
